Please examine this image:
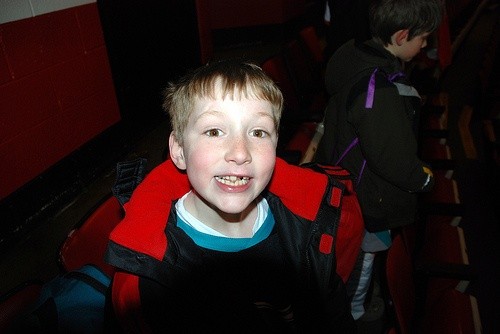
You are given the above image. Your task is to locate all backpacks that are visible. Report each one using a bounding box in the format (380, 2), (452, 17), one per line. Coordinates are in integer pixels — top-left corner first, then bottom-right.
(4, 264), (112, 334)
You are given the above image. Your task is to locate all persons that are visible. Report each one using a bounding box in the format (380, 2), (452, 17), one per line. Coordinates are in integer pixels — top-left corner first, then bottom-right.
(323, 0), (449, 327)
(38, 59), (400, 334)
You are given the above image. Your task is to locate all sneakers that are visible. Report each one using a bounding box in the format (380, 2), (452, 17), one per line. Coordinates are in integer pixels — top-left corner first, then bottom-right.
(357, 297), (385, 321)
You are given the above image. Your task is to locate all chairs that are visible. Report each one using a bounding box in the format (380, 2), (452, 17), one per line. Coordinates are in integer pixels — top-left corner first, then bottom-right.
(0, 22), (500, 334)
